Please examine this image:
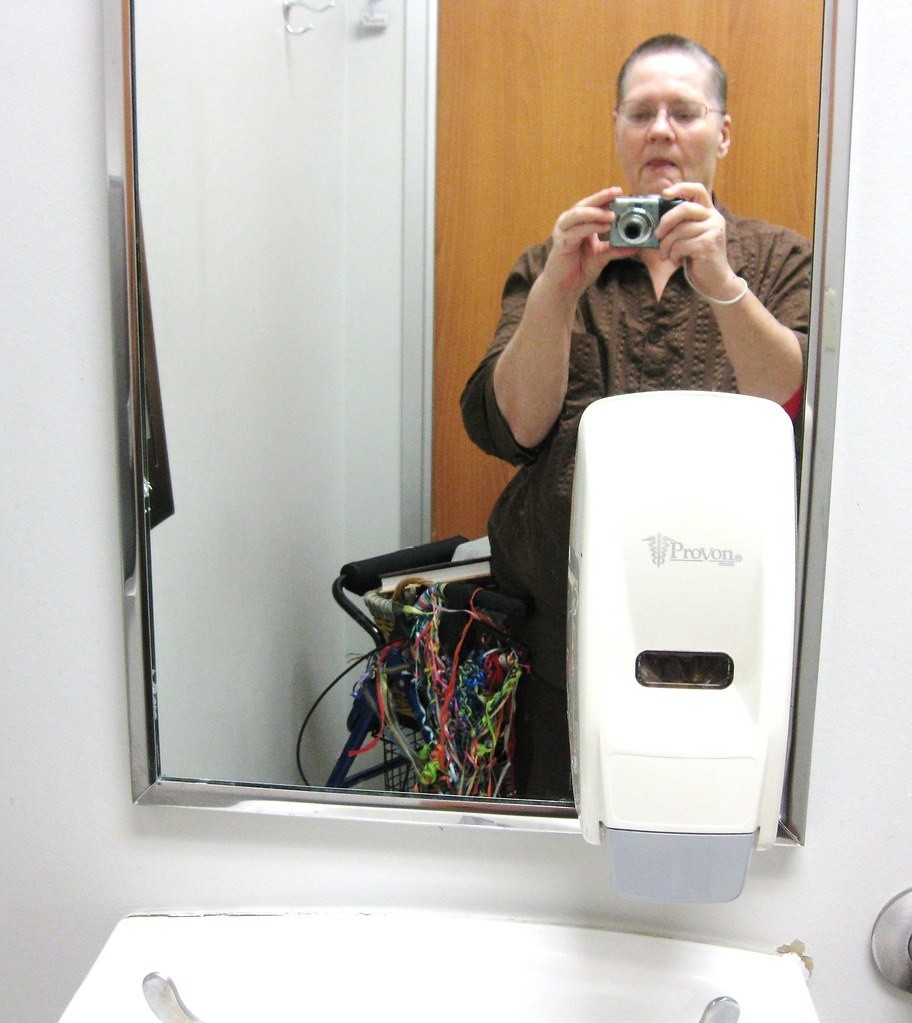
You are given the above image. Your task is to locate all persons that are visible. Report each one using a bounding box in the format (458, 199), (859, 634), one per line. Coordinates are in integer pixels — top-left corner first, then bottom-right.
(458, 30), (814, 806)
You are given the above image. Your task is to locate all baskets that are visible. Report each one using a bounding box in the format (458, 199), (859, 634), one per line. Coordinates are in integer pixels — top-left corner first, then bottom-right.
(364, 578), (509, 719)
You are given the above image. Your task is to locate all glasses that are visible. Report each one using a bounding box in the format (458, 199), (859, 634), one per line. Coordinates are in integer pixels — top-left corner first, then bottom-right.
(617, 99), (724, 128)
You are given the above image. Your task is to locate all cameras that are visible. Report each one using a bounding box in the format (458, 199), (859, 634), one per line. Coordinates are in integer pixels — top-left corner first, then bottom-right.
(610, 195), (686, 249)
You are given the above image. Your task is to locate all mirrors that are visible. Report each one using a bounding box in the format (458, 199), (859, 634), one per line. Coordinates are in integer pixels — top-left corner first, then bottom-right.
(102, 0), (839, 844)
